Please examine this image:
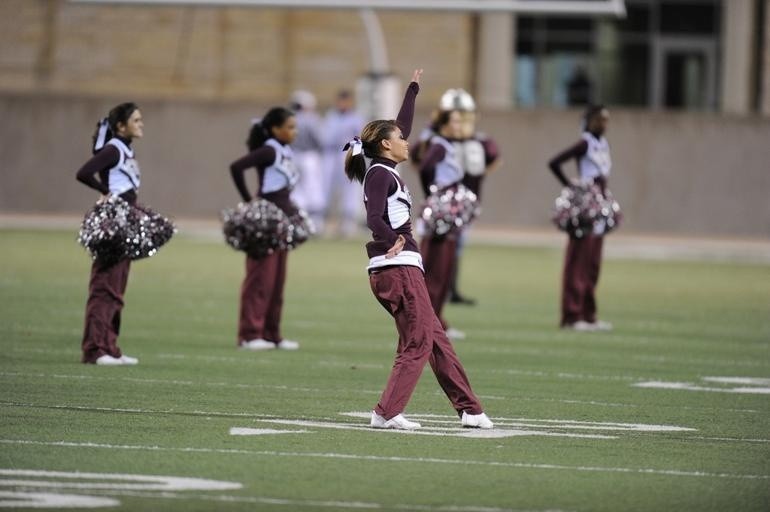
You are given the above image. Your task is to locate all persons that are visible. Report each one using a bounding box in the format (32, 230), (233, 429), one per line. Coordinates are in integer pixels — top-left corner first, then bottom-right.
(549, 102), (615, 331)
(277, 88), (367, 240)
(229, 108), (301, 351)
(76, 101), (145, 366)
(343, 69), (495, 430)
(410, 88), (494, 305)
(421, 111), (467, 341)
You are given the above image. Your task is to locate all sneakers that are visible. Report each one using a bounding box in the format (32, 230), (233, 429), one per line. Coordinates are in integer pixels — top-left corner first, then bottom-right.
(567, 319), (597, 336)
(240, 338), (276, 349)
(370, 409), (422, 430)
(277, 338), (299, 350)
(461, 409), (494, 429)
(118, 355), (138, 365)
(95, 353), (122, 366)
(595, 319), (615, 332)
(446, 326), (469, 342)
(450, 292), (479, 307)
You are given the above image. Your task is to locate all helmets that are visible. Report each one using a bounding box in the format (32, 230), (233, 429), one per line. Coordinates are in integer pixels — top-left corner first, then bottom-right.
(439, 86), (476, 111)
(290, 89), (317, 110)
